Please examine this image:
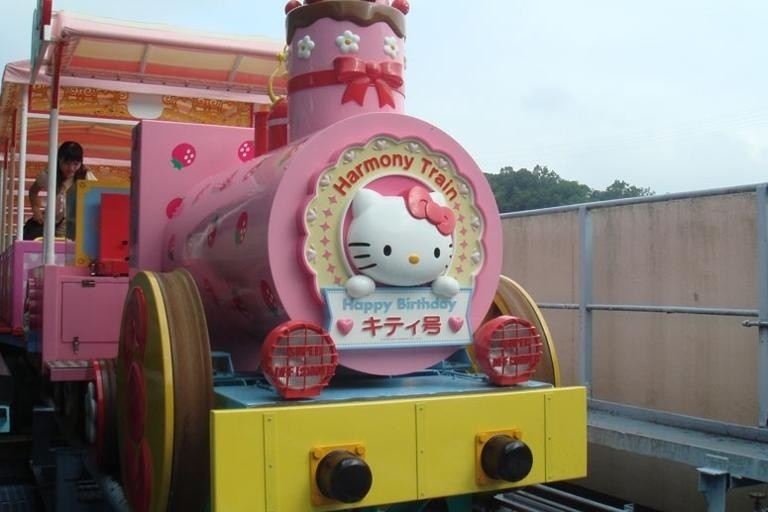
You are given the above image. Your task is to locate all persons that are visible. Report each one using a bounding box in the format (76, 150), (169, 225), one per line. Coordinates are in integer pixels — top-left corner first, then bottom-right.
(23, 211), (45, 241)
(27, 138), (98, 241)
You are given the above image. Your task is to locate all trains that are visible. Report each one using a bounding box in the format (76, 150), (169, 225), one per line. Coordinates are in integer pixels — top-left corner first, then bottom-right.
(0, 0), (590, 511)
